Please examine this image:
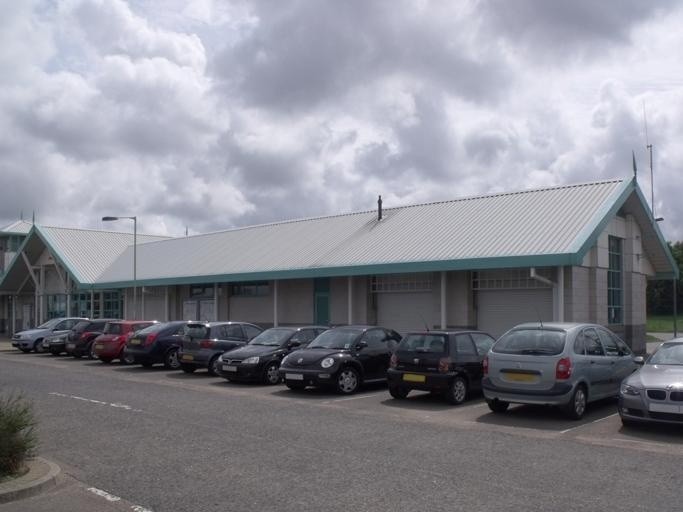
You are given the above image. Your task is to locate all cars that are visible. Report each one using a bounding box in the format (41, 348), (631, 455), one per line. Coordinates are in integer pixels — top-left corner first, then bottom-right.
(93, 319), (164, 367)
(217, 326), (329, 388)
(277, 325), (405, 397)
(66, 317), (120, 360)
(387, 314), (502, 405)
(122, 320), (204, 373)
(41, 332), (69, 356)
(616, 335), (683, 431)
(480, 307), (640, 422)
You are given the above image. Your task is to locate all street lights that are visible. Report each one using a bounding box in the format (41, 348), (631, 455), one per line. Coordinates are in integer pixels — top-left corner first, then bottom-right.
(102, 214), (138, 320)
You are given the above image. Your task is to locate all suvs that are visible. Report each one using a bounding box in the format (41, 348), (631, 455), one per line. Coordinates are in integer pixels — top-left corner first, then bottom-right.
(11, 315), (89, 355)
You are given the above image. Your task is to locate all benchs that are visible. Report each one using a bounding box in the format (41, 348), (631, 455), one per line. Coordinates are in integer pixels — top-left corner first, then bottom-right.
(504, 332), (561, 349)
(411, 339), (443, 349)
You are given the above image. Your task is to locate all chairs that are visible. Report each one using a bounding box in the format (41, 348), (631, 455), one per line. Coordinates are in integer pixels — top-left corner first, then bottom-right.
(349, 335), (357, 344)
(330, 334), (345, 348)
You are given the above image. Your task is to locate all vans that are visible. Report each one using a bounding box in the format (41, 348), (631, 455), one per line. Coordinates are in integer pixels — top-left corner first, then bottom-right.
(179, 320), (264, 375)
